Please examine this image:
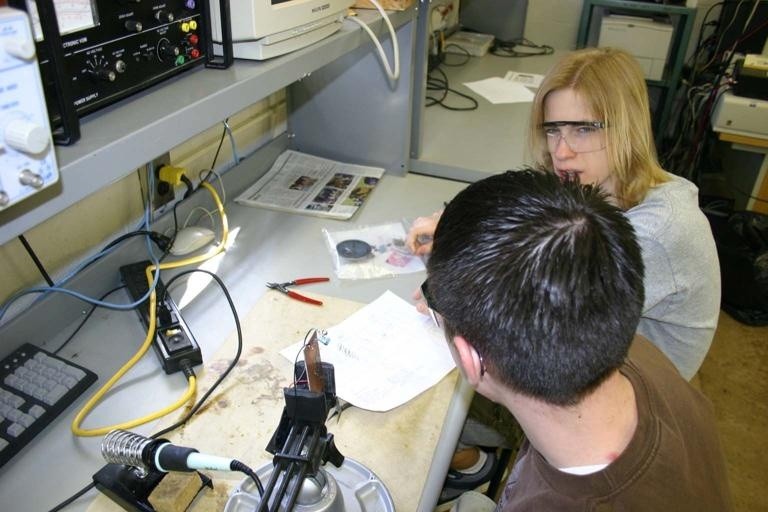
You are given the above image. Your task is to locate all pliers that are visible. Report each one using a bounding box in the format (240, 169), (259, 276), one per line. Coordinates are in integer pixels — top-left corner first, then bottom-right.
(265, 278), (329, 304)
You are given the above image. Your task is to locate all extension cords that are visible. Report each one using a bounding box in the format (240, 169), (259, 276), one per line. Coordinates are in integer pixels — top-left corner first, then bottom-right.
(119, 260), (202, 375)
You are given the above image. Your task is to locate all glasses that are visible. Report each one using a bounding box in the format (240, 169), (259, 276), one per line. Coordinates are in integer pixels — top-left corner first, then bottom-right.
(538, 121), (606, 154)
(419, 281), (484, 376)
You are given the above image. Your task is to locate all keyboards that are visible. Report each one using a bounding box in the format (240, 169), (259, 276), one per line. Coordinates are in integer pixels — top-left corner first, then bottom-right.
(0, 340), (98, 470)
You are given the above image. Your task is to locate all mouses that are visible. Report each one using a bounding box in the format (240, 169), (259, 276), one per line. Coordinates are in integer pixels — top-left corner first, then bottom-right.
(168, 226), (215, 256)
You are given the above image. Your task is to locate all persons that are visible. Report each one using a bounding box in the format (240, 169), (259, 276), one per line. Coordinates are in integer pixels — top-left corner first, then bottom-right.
(411, 167), (733, 512)
(405, 47), (722, 506)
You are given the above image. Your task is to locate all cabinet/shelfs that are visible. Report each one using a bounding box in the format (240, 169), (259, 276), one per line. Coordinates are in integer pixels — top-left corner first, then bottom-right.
(576, 0), (703, 158)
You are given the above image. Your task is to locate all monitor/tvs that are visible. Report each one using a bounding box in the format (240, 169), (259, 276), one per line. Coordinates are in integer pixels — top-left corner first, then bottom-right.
(210, 0), (356, 61)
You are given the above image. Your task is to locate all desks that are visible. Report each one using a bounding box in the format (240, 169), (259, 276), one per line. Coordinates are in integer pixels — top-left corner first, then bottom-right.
(2, 45), (578, 512)
